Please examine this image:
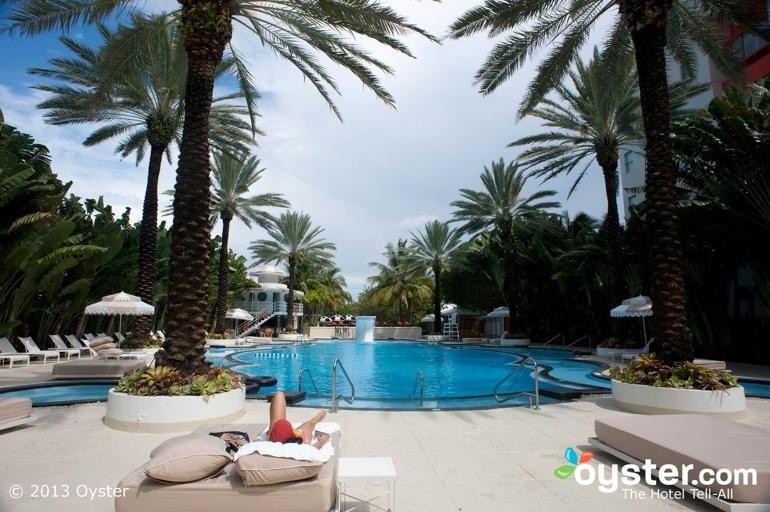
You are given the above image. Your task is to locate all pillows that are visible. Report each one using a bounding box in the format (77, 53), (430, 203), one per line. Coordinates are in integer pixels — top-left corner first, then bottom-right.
(145, 431), (324, 488)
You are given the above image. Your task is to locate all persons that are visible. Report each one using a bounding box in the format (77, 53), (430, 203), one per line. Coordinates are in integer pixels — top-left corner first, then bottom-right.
(375, 318), (413, 327)
(243, 304), (298, 339)
(253, 391), (330, 449)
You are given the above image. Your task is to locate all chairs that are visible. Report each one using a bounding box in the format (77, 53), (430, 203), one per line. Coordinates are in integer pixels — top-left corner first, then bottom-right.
(0, 330), (166, 369)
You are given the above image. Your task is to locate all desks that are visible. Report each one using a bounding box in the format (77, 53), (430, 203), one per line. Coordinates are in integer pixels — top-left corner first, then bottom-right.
(335, 455), (397, 512)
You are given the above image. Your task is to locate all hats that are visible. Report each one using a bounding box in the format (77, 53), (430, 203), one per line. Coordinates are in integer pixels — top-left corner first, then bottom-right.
(268, 420), (301, 444)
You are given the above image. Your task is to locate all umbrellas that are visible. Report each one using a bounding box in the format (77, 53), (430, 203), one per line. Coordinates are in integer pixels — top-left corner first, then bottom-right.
(440, 303), (462, 323)
(331, 314), (345, 321)
(83, 290), (155, 361)
(318, 316), (333, 323)
(421, 313), (444, 333)
(609, 294), (653, 345)
(487, 306), (519, 319)
(225, 306), (254, 338)
(343, 314), (355, 320)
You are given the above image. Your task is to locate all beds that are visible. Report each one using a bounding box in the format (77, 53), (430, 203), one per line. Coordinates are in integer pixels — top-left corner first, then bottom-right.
(50, 359), (147, 379)
(587, 412), (770, 511)
(116, 422), (341, 512)
